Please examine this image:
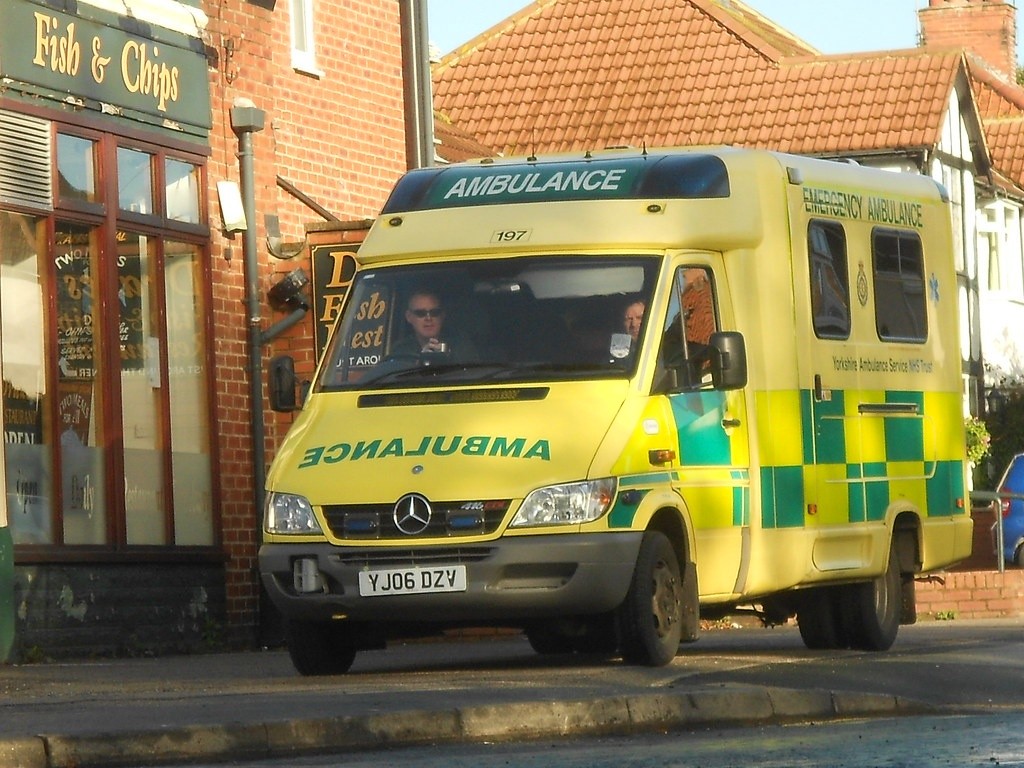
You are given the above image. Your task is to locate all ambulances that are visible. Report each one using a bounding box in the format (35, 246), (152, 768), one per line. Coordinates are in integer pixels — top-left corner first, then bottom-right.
(251, 137), (976, 672)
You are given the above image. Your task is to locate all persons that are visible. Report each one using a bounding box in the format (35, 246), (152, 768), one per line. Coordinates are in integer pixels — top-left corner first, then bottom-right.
(603, 297), (683, 370)
(378, 290), (476, 373)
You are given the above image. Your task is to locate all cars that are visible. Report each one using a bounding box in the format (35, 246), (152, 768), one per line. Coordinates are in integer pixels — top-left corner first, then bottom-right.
(988, 453), (1024, 571)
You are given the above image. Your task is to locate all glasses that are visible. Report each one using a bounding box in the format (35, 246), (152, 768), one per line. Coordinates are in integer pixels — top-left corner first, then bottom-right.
(412, 308), (442, 317)
